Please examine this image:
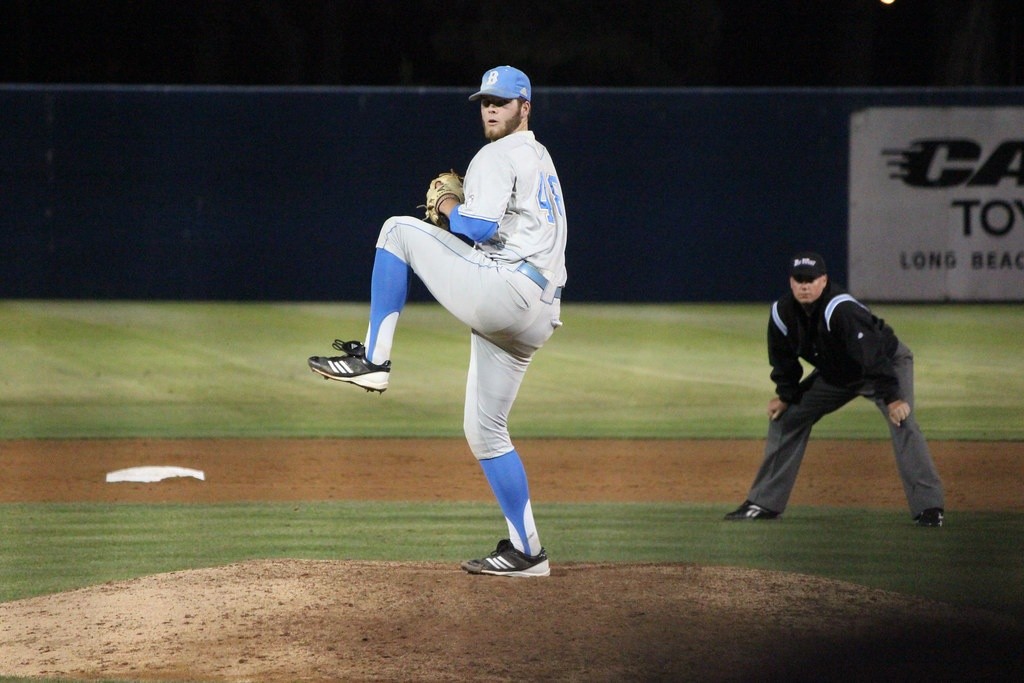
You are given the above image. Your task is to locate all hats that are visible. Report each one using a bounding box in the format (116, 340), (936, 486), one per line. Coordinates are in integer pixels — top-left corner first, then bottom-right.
(469, 66), (531, 102)
(791, 253), (826, 277)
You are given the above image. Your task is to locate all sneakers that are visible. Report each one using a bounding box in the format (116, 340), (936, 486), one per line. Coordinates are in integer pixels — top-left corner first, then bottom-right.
(460, 539), (550, 577)
(725, 500), (780, 520)
(920, 509), (943, 527)
(307, 339), (389, 391)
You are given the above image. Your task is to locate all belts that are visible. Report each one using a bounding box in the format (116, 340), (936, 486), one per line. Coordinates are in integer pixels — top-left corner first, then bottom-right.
(517, 262), (562, 299)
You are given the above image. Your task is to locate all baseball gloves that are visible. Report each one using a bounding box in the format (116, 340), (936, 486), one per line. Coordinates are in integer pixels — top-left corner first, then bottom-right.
(416, 165), (466, 229)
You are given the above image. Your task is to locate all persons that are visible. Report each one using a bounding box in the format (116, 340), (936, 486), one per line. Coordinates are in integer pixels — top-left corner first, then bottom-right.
(724, 251), (944, 529)
(308, 65), (567, 576)
